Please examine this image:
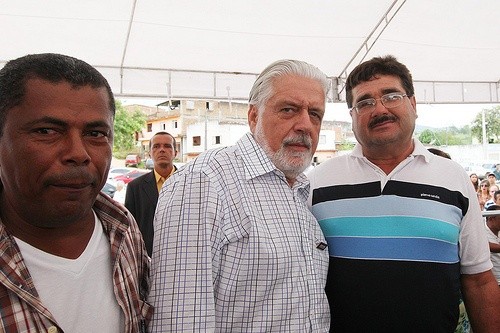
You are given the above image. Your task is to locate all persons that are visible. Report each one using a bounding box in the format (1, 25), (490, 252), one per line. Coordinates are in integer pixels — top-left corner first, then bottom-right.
(0, 53), (152, 333)
(148, 60), (331, 333)
(112, 181), (127, 206)
(125, 132), (177, 257)
(306, 57), (500, 333)
(428, 147), (500, 254)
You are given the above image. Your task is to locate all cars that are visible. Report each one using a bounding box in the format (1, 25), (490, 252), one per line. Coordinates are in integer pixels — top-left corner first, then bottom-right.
(100, 179), (127, 198)
(145, 159), (155, 168)
(125, 154), (141, 168)
(108, 167), (153, 184)
